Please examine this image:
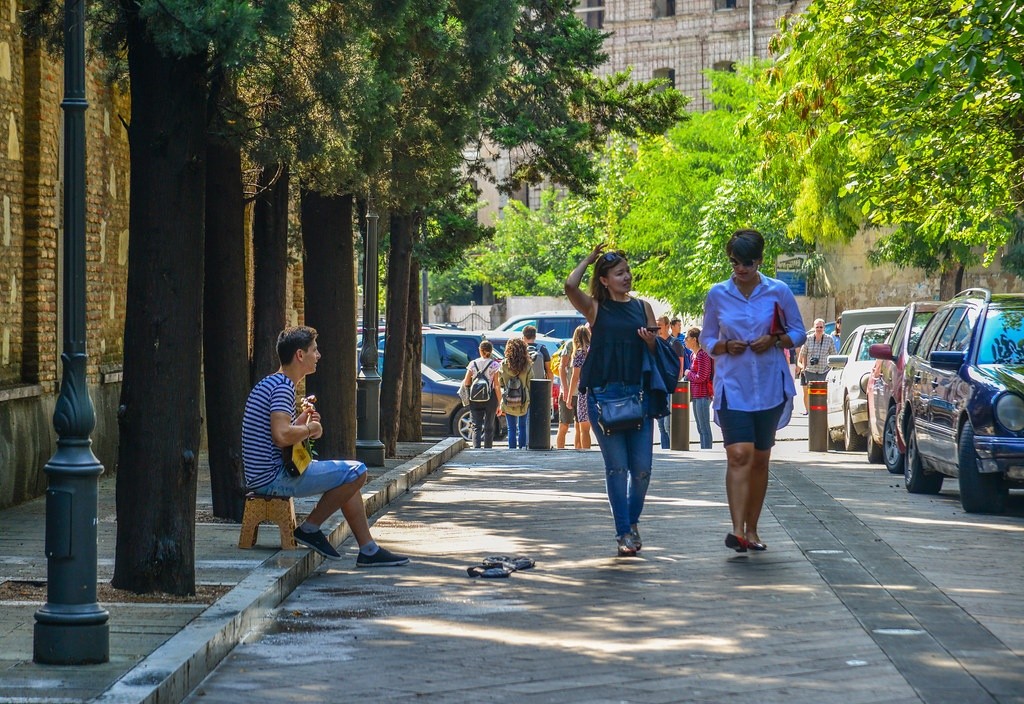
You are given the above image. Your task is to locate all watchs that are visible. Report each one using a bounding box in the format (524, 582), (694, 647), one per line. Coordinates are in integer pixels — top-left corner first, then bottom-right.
(774, 334), (782, 348)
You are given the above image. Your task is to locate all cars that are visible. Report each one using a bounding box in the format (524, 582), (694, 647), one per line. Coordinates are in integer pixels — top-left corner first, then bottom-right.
(355, 349), (510, 444)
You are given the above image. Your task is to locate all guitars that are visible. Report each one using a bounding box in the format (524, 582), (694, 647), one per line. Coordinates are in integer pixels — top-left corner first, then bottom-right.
(283, 394), (317, 478)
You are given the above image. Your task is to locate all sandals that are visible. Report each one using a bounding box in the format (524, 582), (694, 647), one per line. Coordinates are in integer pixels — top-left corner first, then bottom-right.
(614, 538), (638, 557)
(631, 524), (641, 550)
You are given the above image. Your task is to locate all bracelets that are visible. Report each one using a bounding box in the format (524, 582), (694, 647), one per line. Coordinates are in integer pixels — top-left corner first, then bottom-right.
(726, 339), (732, 354)
(304, 424), (311, 439)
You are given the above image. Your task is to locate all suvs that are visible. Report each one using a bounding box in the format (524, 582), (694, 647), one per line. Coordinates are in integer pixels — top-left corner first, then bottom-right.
(862, 299), (946, 475)
(899, 285), (1024, 516)
(822, 322), (896, 454)
(358, 331), (509, 387)
(494, 311), (592, 342)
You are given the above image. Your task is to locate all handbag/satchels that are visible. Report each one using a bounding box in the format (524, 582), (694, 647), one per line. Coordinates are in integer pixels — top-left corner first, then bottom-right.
(596, 395), (646, 435)
(771, 302), (787, 336)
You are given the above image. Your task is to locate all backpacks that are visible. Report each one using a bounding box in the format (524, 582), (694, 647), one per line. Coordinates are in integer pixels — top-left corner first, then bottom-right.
(502, 375), (527, 407)
(468, 360), (493, 402)
(549, 340), (573, 375)
(526, 343), (546, 379)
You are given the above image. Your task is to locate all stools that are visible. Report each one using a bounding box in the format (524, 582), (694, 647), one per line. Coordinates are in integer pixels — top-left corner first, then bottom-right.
(235, 493), (296, 551)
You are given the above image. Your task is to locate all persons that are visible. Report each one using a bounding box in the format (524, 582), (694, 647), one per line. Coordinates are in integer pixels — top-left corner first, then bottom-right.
(241, 326), (412, 569)
(699, 230), (808, 552)
(461, 317), (841, 449)
(565, 240), (659, 556)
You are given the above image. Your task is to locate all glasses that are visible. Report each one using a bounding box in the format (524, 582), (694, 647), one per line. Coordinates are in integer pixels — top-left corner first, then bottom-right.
(816, 326), (823, 328)
(603, 249), (625, 263)
(729, 256), (756, 266)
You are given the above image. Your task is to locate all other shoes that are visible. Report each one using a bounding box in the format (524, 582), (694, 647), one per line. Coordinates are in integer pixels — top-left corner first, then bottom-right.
(726, 534), (747, 552)
(745, 534), (766, 550)
(294, 527), (342, 560)
(356, 546), (408, 567)
